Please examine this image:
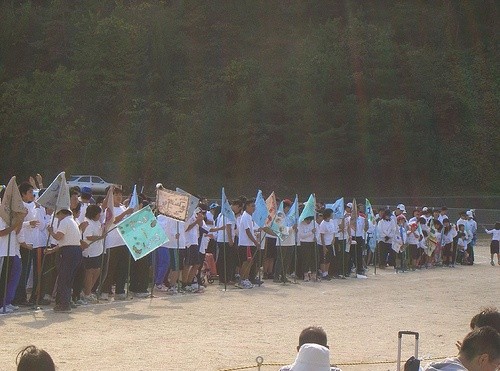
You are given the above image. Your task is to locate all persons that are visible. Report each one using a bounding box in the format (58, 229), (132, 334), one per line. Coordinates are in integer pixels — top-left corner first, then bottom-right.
(15, 345), (56, 371)
(455, 309), (500, 351)
(278, 327), (345, 371)
(423, 327), (500, 371)
(288, 343), (331, 371)
(482, 220), (500, 266)
(0, 173), (478, 314)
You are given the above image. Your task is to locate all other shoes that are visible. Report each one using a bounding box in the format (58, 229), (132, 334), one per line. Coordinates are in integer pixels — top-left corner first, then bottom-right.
(0, 272), (369, 314)
(490, 261), (496, 266)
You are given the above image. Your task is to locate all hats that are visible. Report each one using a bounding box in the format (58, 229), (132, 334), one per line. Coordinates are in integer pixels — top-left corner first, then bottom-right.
(396, 204), (406, 213)
(55, 207), (73, 218)
(289, 343), (330, 371)
(196, 207), (206, 213)
(209, 204), (219, 208)
(372, 209), (379, 214)
(81, 187), (91, 193)
(466, 211), (473, 218)
(422, 207), (428, 211)
(385, 209), (394, 216)
(283, 199), (293, 204)
(397, 215), (406, 221)
(347, 202), (352, 208)
(409, 223), (418, 229)
(441, 207), (449, 210)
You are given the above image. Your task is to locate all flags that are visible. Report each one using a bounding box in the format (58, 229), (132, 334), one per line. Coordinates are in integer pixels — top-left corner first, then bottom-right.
(350, 198), (358, 231)
(176, 187), (199, 220)
(124, 185), (140, 218)
(330, 197), (344, 219)
(1, 176), (29, 234)
(156, 184), (190, 223)
(251, 190), (270, 228)
(114, 206), (169, 261)
(34, 171), (71, 213)
(298, 194), (315, 224)
(103, 183), (115, 233)
(221, 188), (236, 222)
(365, 198), (374, 222)
(271, 201), (289, 242)
(266, 191), (277, 227)
(285, 194), (299, 227)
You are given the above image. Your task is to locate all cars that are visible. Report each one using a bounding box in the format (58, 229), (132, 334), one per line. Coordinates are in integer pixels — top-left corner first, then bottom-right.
(67, 175), (123, 195)
